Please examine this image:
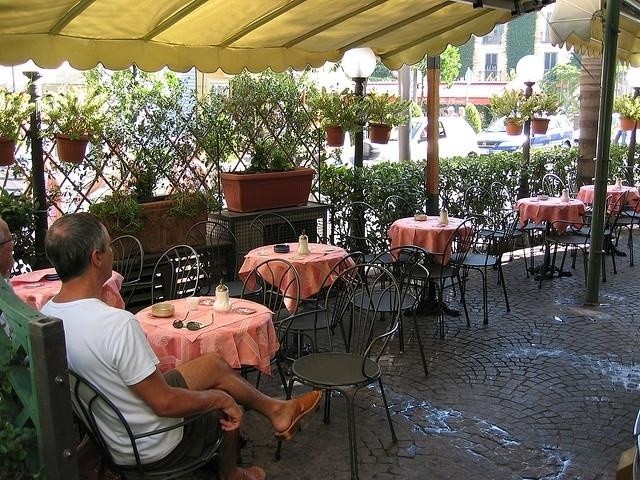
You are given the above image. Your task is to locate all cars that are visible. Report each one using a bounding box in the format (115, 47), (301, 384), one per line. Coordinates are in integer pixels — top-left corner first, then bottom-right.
(570, 112), (623, 150)
(347, 116), (480, 170)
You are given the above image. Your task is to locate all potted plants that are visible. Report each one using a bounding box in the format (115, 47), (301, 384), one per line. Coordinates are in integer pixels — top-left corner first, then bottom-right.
(311, 86), (419, 148)
(218, 72), (317, 208)
(89, 72), (208, 256)
(50, 92), (99, 164)
(489, 86), (561, 135)
(0, 89), (39, 166)
(614, 93), (640, 131)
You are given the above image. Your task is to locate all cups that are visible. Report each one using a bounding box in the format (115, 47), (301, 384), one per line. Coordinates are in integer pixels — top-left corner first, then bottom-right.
(188, 297), (199, 311)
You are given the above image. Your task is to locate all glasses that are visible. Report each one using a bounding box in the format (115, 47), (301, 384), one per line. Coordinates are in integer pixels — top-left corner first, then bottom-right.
(0, 233), (18, 246)
(172, 312), (214, 331)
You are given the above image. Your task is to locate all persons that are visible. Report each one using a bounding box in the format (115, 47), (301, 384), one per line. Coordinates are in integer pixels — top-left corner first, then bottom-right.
(0, 217), (30, 368)
(36, 212), (324, 480)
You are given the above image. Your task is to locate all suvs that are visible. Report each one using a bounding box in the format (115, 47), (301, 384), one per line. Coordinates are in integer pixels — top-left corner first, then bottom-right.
(475, 108), (572, 158)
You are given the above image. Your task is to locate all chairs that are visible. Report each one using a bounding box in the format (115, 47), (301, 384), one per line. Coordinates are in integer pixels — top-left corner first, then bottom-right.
(275, 263), (401, 479)
(67, 369), (243, 479)
(448, 172), (640, 326)
(237, 257), (303, 433)
(1, 194), (450, 377)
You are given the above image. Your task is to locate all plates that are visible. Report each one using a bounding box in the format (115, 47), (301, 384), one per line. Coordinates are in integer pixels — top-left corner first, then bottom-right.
(45, 274), (59, 281)
(198, 298), (215, 306)
(234, 307), (257, 314)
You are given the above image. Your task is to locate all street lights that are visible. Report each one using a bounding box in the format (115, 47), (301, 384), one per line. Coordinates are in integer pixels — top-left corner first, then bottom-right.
(623, 67), (639, 189)
(339, 46), (380, 284)
(515, 53), (548, 226)
(16, 60), (55, 269)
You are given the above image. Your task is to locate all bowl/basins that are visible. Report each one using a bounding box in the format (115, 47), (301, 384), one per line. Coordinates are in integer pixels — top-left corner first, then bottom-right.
(415, 215), (428, 221)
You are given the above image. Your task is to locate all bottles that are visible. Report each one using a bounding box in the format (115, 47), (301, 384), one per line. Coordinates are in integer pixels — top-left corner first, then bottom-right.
(213, 278), (232, 314)
(440, 205), (449, 225)
(561, 186), (570, 203)
(296, 229), (311, 255)
(614, 177), (624, 191)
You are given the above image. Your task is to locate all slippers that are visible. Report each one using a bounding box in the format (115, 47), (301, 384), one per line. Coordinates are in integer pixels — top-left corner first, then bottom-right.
(275, 390), (323, 441)
(239, 466), (266, 480)
